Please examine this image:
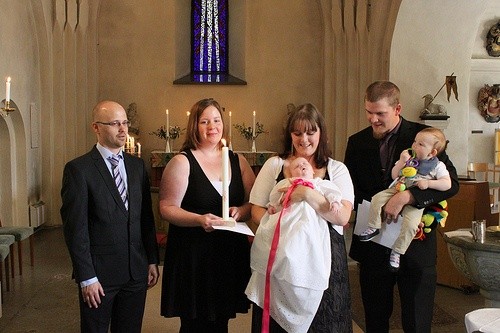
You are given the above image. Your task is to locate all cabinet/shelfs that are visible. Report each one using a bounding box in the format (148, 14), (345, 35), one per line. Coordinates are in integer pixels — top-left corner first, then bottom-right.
(469, 165), (500, 204)
(436, 180), (490, 294)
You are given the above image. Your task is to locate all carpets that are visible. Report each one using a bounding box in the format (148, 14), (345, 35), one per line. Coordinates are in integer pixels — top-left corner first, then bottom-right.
(341, 264), (484, 333)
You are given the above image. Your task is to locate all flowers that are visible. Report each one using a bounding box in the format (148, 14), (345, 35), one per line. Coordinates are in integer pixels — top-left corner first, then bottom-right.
(238, 122), (264, 140)
(155, 125), (180, 140)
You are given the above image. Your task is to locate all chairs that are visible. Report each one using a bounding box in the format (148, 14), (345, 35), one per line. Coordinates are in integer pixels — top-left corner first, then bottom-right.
(0, 244), (10, 302)
(467, 162), (494, 206)
(0, 220), (34, 276)
(0, 235), (15, 280)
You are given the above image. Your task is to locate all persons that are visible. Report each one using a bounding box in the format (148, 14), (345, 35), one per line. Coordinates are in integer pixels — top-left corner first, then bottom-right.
(247, 101), (356, 333)
(158, 98), (259, 333)
(358, 126), (453, 272)
(243, 156), (343, 332)
(341, 79), (462, 333)
(60, 100), (160, 333)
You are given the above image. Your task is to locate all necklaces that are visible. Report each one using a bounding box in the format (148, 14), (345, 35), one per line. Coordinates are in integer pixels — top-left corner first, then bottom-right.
(293, 153), (316, 163)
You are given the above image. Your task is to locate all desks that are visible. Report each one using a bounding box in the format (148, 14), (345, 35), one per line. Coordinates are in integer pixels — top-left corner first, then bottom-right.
(444, 227), (500, 308)
(151, 150), (276, 190)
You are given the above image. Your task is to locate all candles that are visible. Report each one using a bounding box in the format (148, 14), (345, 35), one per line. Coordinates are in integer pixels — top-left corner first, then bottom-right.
(229, 112), (232, 139)
(5, 76), (12, 102)
(126, 135), (141, 153)
(221, 139), (229, 220)
(252, 111), (256, 137)
(166, 109), (170, 135)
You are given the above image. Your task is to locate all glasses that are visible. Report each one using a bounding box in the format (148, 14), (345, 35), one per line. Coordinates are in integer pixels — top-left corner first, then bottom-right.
(96, 120), (131, 126)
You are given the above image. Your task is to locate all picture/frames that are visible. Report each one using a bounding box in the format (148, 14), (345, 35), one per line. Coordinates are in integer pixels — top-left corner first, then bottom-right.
(30, 104), (40, 148)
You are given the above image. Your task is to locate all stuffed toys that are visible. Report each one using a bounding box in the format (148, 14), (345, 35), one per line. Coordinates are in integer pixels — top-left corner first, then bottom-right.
(420, 199), (449, 234)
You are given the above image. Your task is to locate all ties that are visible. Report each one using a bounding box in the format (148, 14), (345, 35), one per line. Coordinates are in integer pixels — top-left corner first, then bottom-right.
(108, 156), (128, 210)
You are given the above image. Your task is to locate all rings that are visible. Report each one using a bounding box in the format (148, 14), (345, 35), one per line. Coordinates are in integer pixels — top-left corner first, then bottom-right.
(387, 217), (391, 220)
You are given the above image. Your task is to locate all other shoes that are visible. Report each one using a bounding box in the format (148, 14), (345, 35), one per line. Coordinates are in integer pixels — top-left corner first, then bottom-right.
(387, 250), (401, 272)
(360, 228), (379, 242)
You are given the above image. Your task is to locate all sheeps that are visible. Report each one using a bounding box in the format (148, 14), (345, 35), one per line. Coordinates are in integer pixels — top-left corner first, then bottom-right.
(421, 94), (447, 116)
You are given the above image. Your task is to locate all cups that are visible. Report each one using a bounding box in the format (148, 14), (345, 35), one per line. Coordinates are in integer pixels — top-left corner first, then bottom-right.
(469, 219), (488, 244)
